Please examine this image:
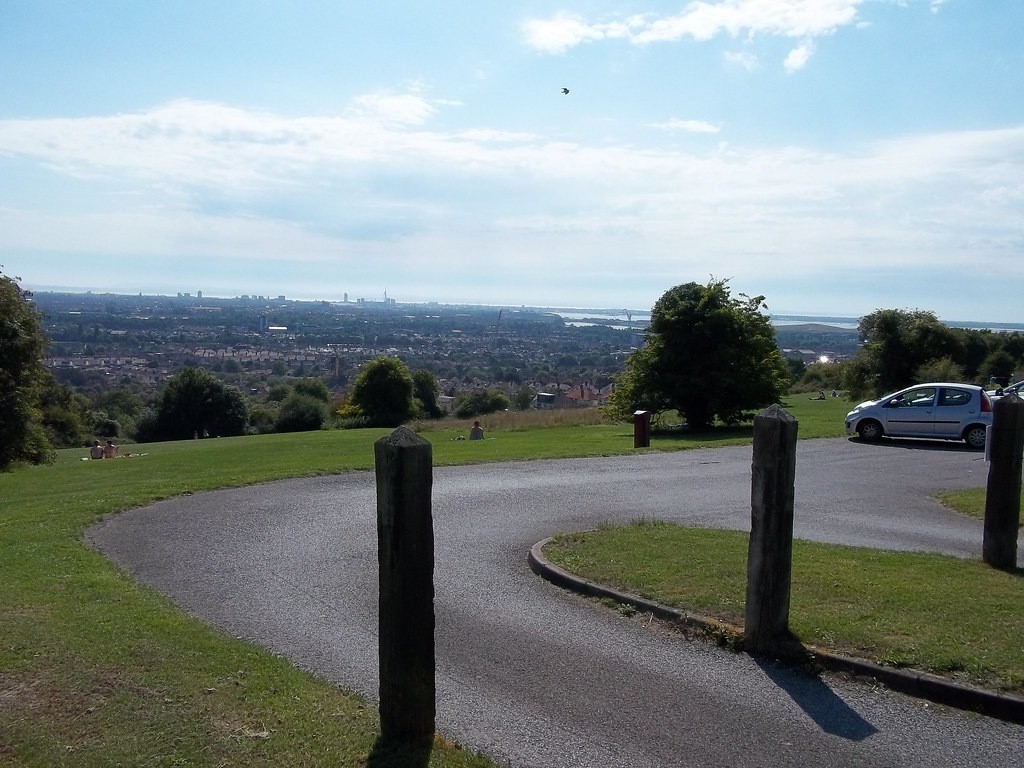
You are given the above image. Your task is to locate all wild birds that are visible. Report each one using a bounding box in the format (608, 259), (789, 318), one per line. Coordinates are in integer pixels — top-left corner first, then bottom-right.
(561, 87), (570, 95)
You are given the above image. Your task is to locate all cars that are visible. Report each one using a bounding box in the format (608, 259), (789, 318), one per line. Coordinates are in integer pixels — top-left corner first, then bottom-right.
(844, 379), (1024, 450)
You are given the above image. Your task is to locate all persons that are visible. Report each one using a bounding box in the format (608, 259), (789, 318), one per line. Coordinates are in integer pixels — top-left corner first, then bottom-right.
(89, 439), (119, 459)
(1011, 374), (1016, 384)
(990, 375), (996, 389)
(818, 390), (825, 400)
(831, 390), (836, 397)
(193, 426), (209, 440)
(469, 421), (485, 441)
